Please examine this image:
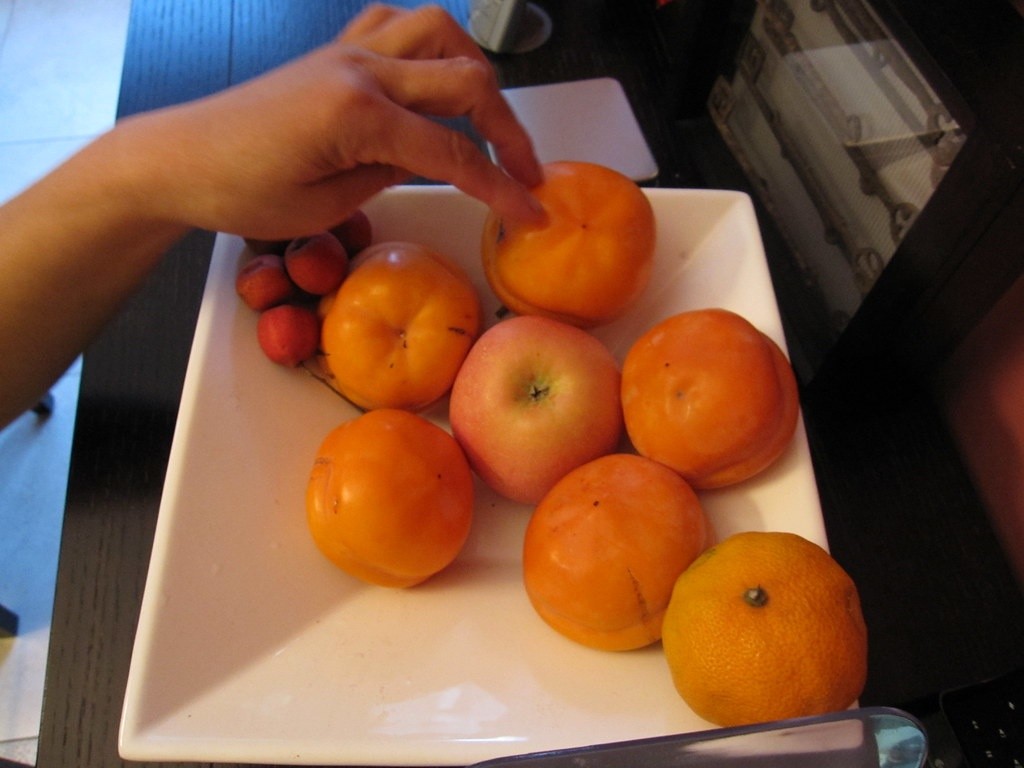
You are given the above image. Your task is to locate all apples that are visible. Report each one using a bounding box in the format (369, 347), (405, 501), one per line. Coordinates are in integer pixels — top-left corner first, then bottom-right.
(448, 316), (622, 505)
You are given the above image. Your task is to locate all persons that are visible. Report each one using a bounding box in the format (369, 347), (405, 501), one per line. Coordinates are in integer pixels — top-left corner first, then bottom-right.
(0, 2), (547, 432)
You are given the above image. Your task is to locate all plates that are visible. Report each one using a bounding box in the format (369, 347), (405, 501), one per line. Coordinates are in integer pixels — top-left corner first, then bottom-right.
(119, 181), (867, 765)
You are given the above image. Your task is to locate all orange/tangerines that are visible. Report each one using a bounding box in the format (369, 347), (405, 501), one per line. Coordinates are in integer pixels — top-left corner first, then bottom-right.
(660, 533), (868, 729)
